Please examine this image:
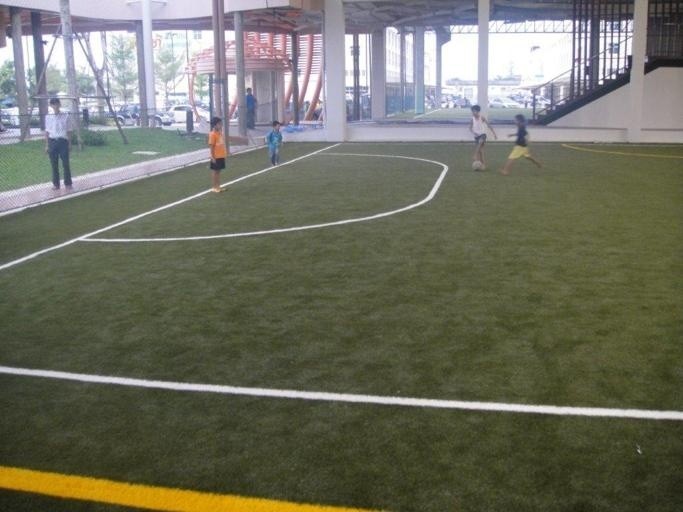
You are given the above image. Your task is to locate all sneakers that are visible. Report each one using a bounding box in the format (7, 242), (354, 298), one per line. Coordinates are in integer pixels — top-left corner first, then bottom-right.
(51, 185), (74, 190)
(211, 186), (226, 193)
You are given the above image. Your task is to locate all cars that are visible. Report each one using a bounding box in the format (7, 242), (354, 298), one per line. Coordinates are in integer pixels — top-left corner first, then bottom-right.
(103, 101), (211, 127)
(489, 90), (550, 109)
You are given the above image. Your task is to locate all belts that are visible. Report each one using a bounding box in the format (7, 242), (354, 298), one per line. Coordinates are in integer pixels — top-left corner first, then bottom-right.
(48, 138), (65, 141)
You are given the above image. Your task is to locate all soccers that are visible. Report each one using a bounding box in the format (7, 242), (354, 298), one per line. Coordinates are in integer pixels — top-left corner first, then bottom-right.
(473, 160), (482, 171)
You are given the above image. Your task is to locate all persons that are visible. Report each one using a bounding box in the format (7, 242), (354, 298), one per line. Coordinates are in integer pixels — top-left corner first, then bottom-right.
(245, 87), (258, 131)
(266, 121), (283, 167)
(43, 97), (76, 191)
(209, 117), (226, 193)
(501, 114), (544, 175)
(469, 104), (497, 170)
